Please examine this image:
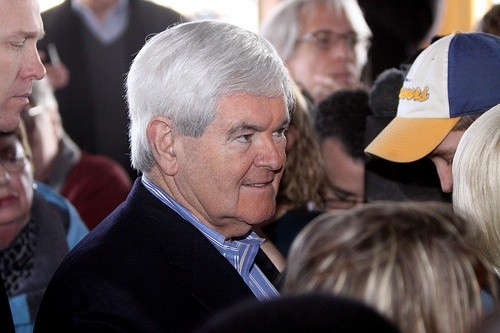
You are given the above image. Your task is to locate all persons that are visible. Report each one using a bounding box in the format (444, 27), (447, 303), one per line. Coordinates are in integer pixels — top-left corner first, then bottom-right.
(364, 30), (500, 193)
(20, 77), (130, 231)
(202, 292), (399, 333)
(453, 103), (500, 275)
(249, 1), (440, 251)
(0, 117), (94, 333)
(280, 201), (486, 332)
(478, 6), (500, 39)
(37, 1), (199, 177)
(33, 18), (297, 332)
(0, 0), (47, 134)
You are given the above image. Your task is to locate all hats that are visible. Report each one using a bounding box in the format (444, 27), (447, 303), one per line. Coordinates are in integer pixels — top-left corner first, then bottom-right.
(364, 31), (500, 162)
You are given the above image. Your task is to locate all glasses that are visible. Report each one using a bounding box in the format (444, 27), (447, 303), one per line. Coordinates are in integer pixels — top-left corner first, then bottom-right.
(295, 28), (358, 49)
(0, 144), (30, 172)
(320, 199), (372, 209)
